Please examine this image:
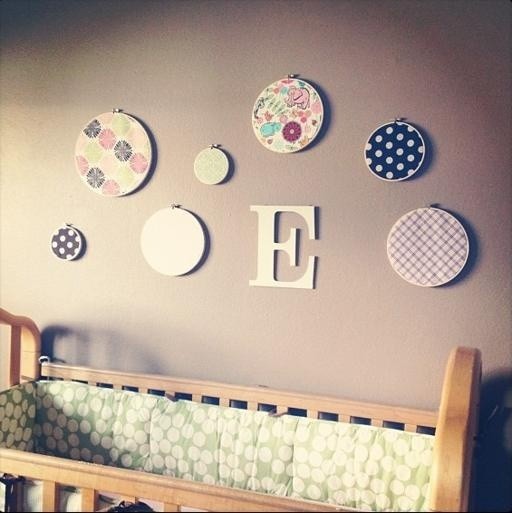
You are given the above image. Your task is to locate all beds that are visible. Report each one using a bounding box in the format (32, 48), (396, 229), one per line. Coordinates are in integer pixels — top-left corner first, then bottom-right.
(1, 306), (481, 513)
